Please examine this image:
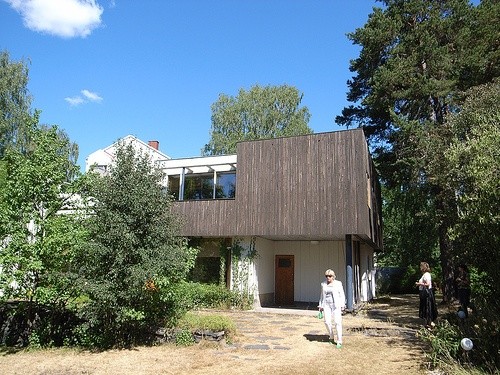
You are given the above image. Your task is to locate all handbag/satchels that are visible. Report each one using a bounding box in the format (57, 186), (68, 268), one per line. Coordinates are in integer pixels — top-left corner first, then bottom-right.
(318, 310), (323, 319)
(421, 282), (430, 298)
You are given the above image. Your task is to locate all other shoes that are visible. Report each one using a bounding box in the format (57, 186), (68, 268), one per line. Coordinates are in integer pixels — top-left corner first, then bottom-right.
(336, 343), (342, 349)
(329, 339), (333, 344)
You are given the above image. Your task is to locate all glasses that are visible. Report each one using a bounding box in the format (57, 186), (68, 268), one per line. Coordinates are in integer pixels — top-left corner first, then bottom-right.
(325, 275), (332, 278)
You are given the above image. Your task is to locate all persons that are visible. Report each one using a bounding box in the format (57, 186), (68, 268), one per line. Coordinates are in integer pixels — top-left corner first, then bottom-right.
(414, 262), (439, 323)
(318, 268), (345, 347)
(455, 263), (472, 319)
